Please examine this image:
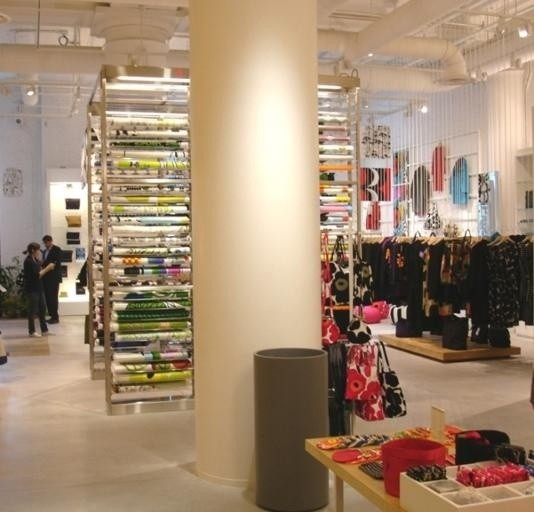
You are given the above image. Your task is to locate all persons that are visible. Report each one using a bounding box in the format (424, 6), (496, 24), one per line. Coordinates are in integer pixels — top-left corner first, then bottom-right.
(42, 235), (63, 324)
(23, 242), (56, 338)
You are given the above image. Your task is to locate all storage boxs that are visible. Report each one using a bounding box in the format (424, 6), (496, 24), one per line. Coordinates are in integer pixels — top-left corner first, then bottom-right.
(376, 437), (448, 498)
(396, 460), (534, 512)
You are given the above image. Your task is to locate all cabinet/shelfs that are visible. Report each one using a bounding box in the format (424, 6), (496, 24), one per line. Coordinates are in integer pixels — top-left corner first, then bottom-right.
(92, 61), (195, 415)
(510, 140), (534, 237)
(316, 66), (359, 344)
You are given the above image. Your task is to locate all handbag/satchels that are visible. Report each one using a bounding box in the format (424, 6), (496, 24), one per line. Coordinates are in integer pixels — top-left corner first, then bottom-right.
(344, 363), (407, 421)
(351, 260), (375, 305)
(321, 318), (340, 345)
(346, 319), (372, 343)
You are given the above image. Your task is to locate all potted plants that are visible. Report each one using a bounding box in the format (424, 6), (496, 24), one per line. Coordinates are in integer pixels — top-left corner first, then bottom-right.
(1, 256), (44, 334)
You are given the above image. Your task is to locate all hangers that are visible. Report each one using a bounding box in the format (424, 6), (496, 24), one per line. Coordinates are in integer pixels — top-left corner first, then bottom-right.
(358, 228), (534, 254)
(437, 140), (444, 149)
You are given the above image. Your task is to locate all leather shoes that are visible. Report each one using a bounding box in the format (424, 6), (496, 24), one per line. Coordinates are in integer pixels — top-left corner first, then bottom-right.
(45, 317), (59, 323)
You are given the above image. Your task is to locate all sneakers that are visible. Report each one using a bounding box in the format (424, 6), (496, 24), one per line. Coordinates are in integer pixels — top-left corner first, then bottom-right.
(29, 332), (41, 337)
(42, 332), (56, 336)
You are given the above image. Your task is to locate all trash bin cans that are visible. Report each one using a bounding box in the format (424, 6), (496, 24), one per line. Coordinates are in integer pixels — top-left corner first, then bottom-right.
(255, 348), (328, 512)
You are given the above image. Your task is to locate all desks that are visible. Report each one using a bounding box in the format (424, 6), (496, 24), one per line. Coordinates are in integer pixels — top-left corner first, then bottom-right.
(303, 424), (534, 512)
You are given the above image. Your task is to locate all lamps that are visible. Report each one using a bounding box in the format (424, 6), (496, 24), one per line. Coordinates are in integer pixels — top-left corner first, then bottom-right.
(489, 15), (532, 37)
